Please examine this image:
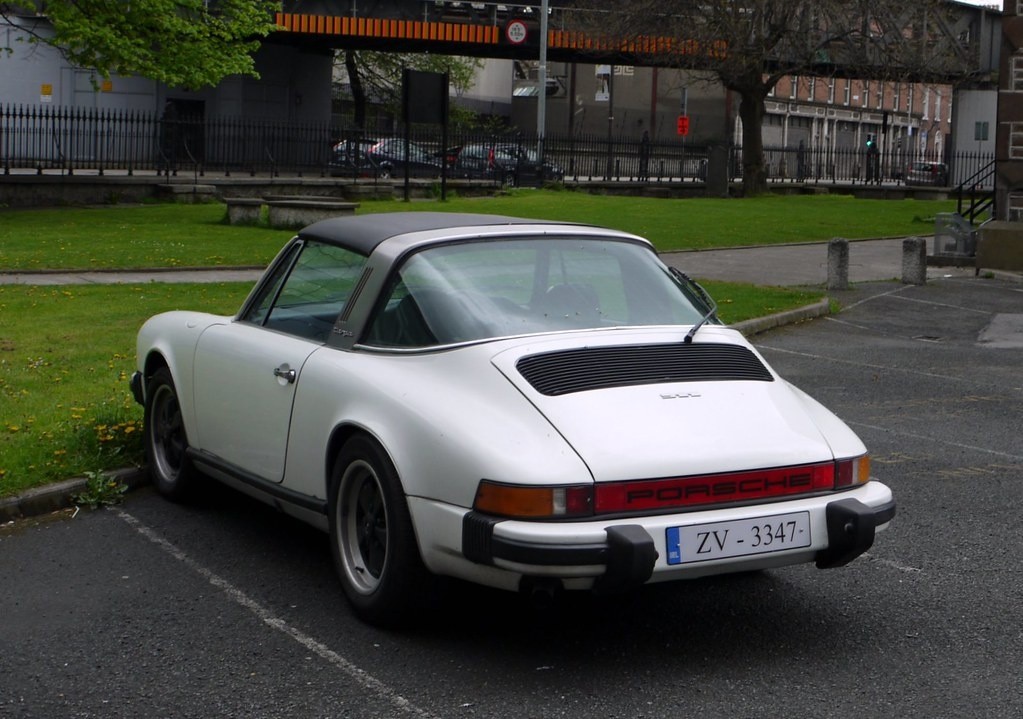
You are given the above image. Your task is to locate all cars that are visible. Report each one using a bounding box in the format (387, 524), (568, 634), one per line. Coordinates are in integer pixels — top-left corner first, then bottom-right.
(434, 144), (565, 187)
(327, 135), (448, 180)
(128, 214), (897, 633)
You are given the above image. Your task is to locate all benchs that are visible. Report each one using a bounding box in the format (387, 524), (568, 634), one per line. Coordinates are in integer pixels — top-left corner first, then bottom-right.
(156, 183), (360, 227)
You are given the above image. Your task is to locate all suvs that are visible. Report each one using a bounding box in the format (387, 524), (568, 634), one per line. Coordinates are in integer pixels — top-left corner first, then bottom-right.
(905, 161), (949, 186)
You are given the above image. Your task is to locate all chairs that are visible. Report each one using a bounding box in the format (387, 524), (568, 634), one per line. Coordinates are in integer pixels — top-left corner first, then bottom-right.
(371, 283), (603, 346)
